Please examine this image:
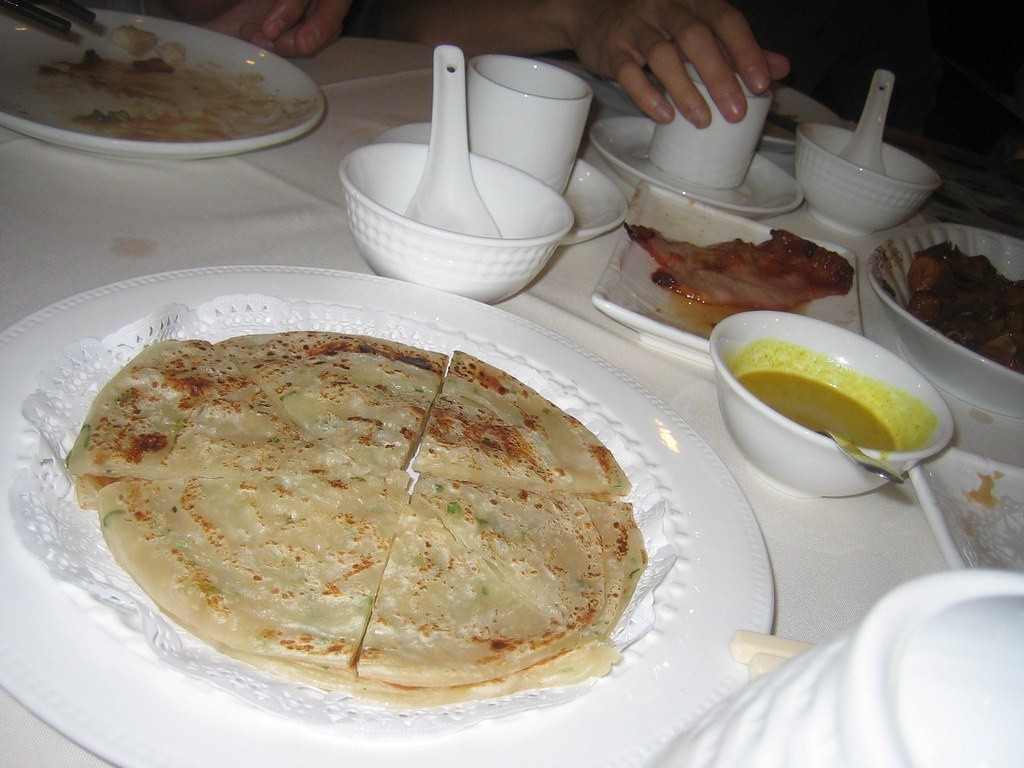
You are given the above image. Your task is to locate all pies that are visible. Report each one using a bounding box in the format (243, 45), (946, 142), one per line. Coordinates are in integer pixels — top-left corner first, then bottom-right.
(65, 329), (648, 708)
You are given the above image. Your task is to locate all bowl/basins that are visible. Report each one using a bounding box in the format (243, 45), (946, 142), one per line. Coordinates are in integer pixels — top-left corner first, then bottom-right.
(795, 122), (942, 237)
(866, 223), (1024, 419)
(709, 310), (954, 500)
(338, 143), (576, 304)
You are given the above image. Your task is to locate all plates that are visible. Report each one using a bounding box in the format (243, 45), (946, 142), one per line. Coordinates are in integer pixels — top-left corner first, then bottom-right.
(0, 265), (773, 768)
(590, 179), (864, 367)
(757, 78), (844, 155)
(372, 122), (630, 246)
(907, 446), (1024, 570)
(588, 116), (804, 218)
(0, 7), (325, 160)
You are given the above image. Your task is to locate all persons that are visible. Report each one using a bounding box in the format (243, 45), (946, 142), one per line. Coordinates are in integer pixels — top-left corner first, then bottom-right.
(80, 1), (1024, 172)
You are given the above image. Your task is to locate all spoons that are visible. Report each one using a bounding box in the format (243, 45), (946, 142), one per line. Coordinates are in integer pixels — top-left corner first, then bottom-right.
(835, 69), (895, 175)
(402, 44), (503, 240)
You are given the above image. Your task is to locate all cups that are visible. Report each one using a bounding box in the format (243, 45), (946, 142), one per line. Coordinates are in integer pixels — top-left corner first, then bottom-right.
(648, 62), (774, 188)
(645, 569), (1024, 768)
(465, 54), (593, 197)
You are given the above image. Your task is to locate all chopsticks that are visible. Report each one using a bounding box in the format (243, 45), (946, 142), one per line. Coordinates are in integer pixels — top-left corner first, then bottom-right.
(0, 0), (96, 34)
(728, 629), (817, 683)
(766, 105), (799, 133)
